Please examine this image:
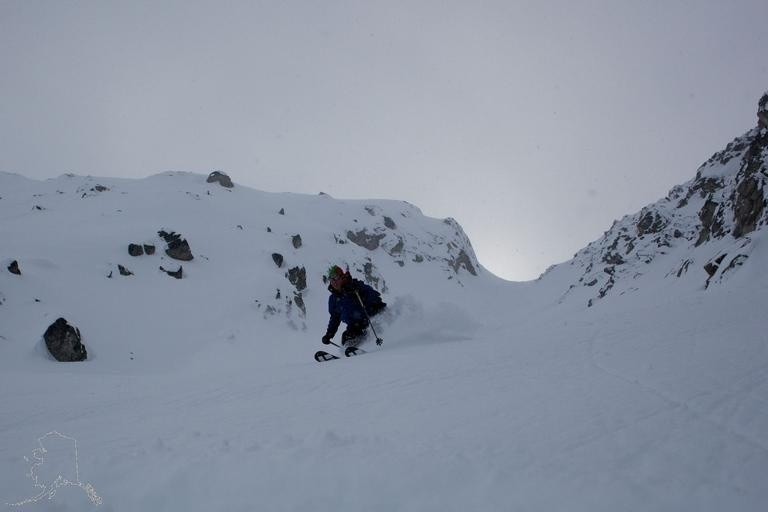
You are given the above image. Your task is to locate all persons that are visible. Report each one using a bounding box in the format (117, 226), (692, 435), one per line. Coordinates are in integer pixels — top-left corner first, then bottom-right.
(322, 264), (387, 352)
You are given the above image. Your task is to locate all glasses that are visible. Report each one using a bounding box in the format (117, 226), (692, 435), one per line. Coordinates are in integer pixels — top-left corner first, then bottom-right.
(328, 275), (341, 281)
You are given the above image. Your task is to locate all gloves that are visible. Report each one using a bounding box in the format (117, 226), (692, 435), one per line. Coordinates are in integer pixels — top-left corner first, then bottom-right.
(322, 330), (334, 344)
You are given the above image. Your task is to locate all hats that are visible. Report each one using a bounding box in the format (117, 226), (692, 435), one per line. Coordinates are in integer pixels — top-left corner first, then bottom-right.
(327, 265), (344, 280)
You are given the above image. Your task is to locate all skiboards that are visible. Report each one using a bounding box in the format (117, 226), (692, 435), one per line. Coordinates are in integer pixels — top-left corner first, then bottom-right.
(314, 346), (368, 362)
